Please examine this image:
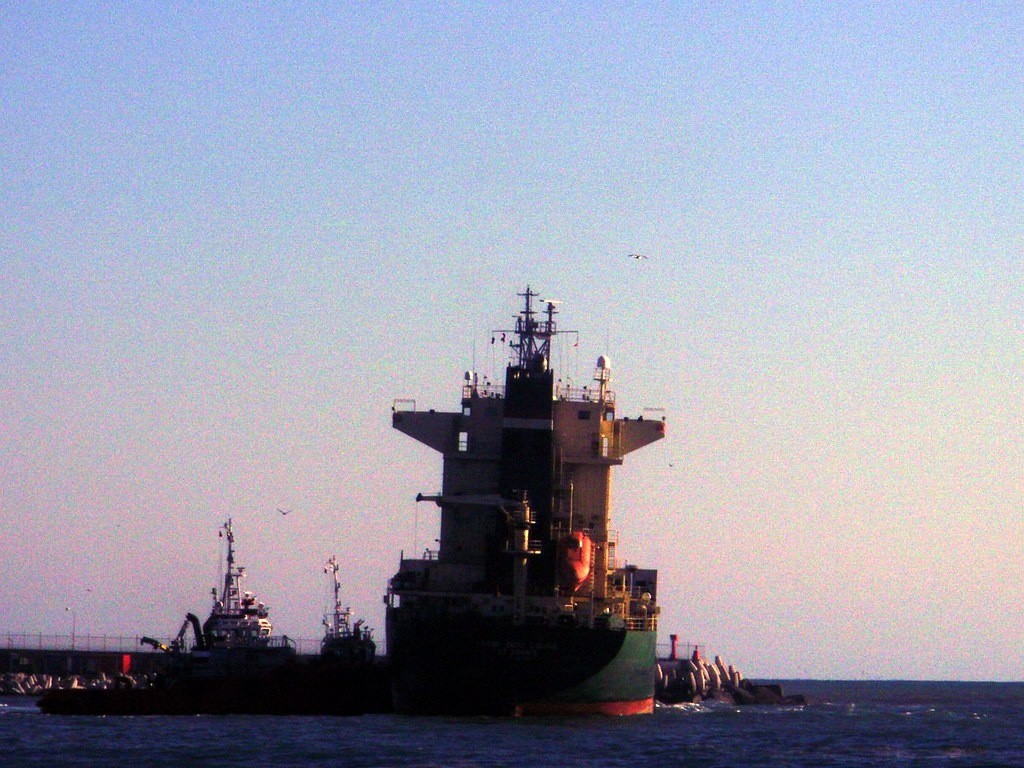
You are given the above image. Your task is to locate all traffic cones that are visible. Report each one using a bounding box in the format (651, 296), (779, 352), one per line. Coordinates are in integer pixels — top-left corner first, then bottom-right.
(692, 645), (701, 661)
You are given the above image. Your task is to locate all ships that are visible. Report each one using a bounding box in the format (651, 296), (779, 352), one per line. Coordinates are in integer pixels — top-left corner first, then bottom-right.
(381, 282), (666, 719)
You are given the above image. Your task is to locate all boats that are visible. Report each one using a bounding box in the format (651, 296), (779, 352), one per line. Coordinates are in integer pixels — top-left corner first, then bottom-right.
(320, 553), (377, 661)
(194, 517), (297, 650)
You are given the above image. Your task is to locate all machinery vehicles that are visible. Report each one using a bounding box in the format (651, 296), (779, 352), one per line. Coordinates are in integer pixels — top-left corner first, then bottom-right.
(139, 612), (208, 654)
